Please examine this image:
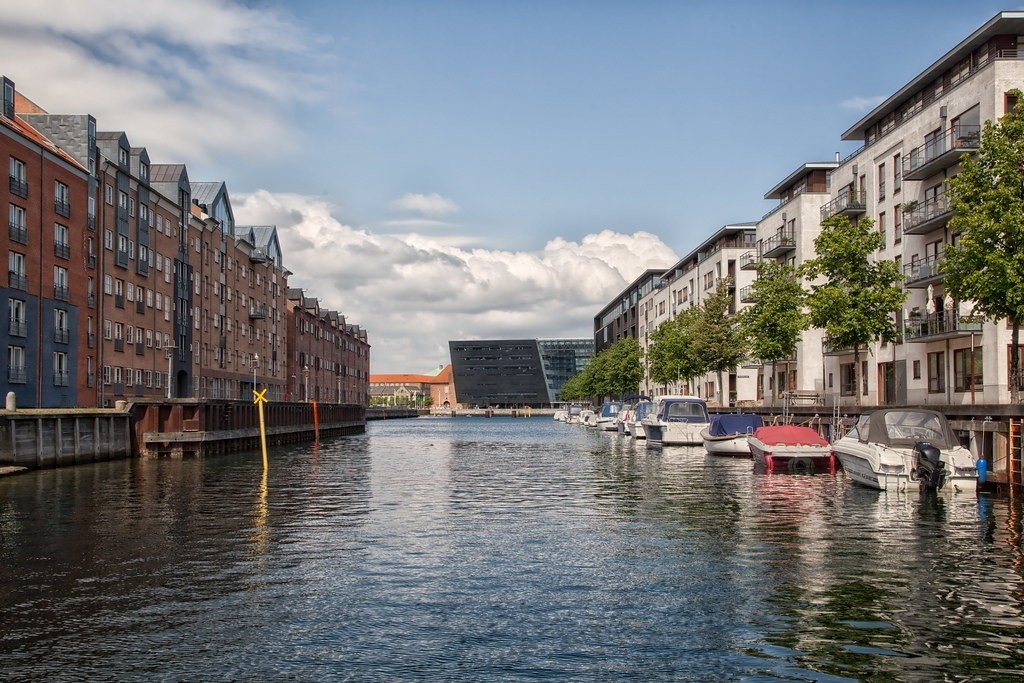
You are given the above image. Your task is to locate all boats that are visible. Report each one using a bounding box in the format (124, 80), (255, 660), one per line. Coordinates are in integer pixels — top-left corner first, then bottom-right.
(747, 424), (835, 478)
(701, 411), (764, 458)
(838, 408), (988, 495)
(554, 395), (710, 458)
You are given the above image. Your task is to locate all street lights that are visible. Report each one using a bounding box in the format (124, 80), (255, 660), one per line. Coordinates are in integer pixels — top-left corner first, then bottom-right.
(252, 351), (261, 400)
(302, 365), (310, 403)
